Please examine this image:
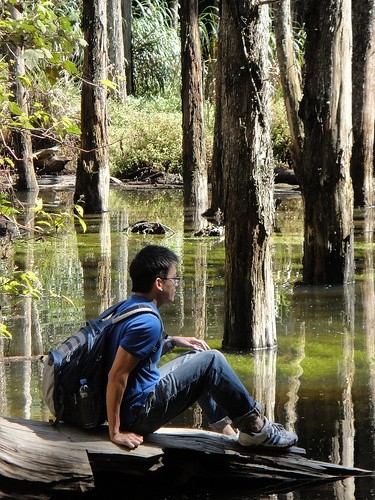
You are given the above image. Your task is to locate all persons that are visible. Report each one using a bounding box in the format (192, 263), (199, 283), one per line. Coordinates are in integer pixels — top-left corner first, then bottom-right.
(94, 245), (297, 451)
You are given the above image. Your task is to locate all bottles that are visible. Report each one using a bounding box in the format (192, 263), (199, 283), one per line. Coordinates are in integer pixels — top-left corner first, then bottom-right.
(78, 378), (96, 430)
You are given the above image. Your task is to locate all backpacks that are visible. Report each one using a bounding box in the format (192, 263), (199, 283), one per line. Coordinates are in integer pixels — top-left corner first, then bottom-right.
(41, 299), (170, 431)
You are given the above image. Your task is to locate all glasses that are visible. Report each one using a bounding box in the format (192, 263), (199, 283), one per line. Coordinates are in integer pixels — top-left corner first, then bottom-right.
(160, 274), (180, 282)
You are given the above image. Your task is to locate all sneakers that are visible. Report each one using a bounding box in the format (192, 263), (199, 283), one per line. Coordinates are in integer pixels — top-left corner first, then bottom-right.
(234, 415), (298, 448)
